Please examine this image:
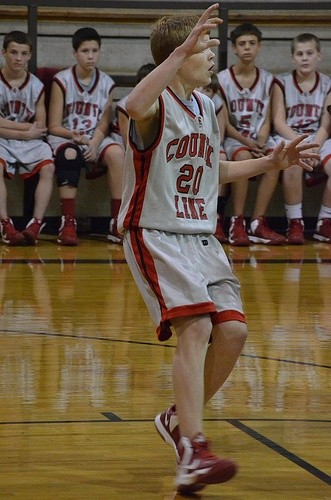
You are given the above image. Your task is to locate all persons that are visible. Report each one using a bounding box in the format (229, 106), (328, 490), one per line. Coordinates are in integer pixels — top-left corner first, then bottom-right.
(0, 23), (331, 246)
(117, 4), (320, 497)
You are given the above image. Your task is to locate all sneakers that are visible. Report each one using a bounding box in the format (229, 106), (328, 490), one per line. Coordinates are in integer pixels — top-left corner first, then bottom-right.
(22, 217), (46, 245)
(175, 433), (236, 493)
(248, 216), (285, 245)
(284, 218), (305, 245)
(0, 217), (26, 246)
(228, 215), (251, 246)
(57, 215), (79, 245)
(214, 214), (228, 243)
(154, 405), (180, 464)
(312, 219), (331, 243)
(107, 218), (123, 244)
